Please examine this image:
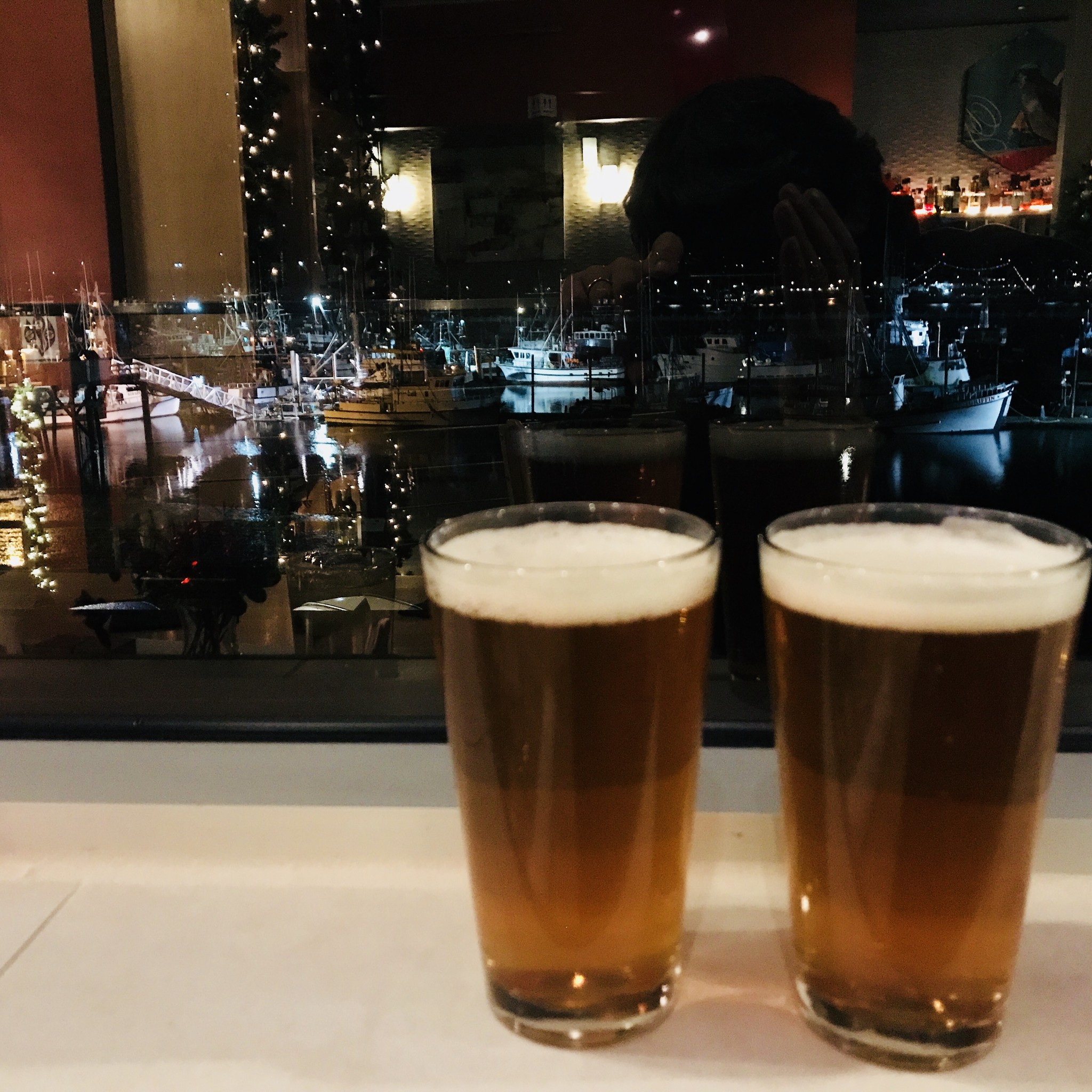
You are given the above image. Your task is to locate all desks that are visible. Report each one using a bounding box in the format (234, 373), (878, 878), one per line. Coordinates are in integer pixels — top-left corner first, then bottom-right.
(2, 739), (1092, 1092)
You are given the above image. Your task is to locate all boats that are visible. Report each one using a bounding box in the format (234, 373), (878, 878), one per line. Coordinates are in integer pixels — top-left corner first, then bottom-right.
(2, 266), (1090, 439)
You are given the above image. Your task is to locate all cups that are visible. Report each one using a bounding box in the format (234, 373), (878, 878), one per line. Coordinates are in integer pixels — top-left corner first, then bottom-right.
(419, 504), (720, 1049)
(758, 503), (1092, 1076)
